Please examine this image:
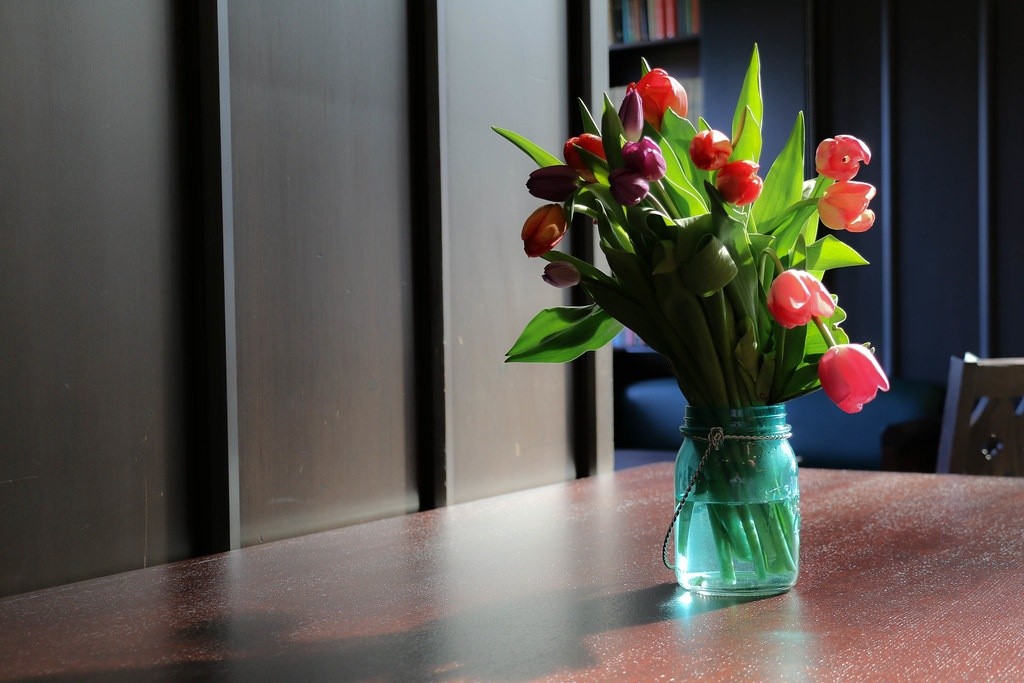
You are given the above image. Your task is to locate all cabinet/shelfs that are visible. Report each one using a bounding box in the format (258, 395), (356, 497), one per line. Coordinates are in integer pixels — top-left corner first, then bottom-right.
(609, 33), (700, 377)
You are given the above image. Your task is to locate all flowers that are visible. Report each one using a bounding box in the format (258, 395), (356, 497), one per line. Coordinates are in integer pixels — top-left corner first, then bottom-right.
(487, 43), (890, 415)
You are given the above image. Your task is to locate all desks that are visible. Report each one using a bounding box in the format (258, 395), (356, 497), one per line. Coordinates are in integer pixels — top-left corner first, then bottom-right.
(0, 461), (1024, 683)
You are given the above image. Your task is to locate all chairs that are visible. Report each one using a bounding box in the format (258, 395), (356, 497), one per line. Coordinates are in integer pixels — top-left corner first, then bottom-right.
(949, 349), (1024, 477)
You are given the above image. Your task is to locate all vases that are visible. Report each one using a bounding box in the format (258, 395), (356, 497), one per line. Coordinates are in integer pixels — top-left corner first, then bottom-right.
(673, 401), (801, 599)
